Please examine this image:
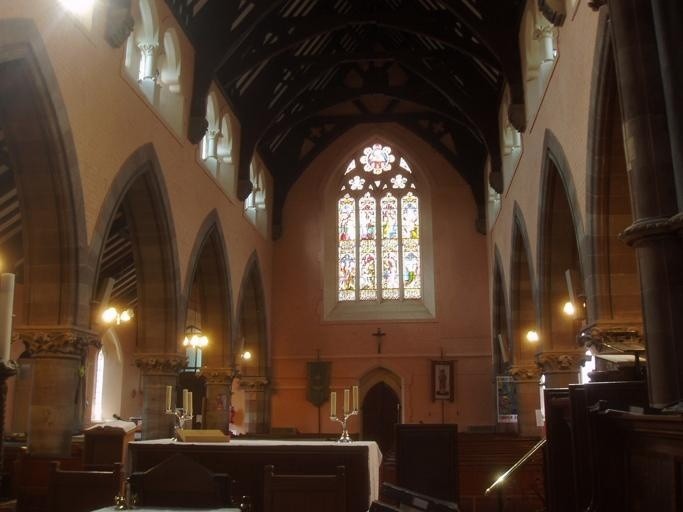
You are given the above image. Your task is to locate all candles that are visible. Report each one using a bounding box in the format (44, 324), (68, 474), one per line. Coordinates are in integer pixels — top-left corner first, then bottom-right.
(331, 392), (336, 415)
(352, 386), (358, 408)
(188, 392), (193, 411)
(344, 390), (349, 413)
(183, 388), (188, 409)
(165, 385), (172, 410)
(0, 274), (16, 362)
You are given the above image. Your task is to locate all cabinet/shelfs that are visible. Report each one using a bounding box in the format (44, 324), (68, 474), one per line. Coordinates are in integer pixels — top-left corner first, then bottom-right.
(83, 420), (136, 497)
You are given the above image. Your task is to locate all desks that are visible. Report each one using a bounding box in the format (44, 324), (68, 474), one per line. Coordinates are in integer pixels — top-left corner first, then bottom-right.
(128, 439), (382, 512)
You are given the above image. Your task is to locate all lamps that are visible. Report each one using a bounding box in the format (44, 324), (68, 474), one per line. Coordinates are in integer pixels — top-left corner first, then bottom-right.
(89, 300), (131, 329)
(183, 325), (208, 348)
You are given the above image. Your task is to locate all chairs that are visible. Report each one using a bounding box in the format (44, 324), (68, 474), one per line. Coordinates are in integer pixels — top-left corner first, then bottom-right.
(262, 464), (346, 512)
